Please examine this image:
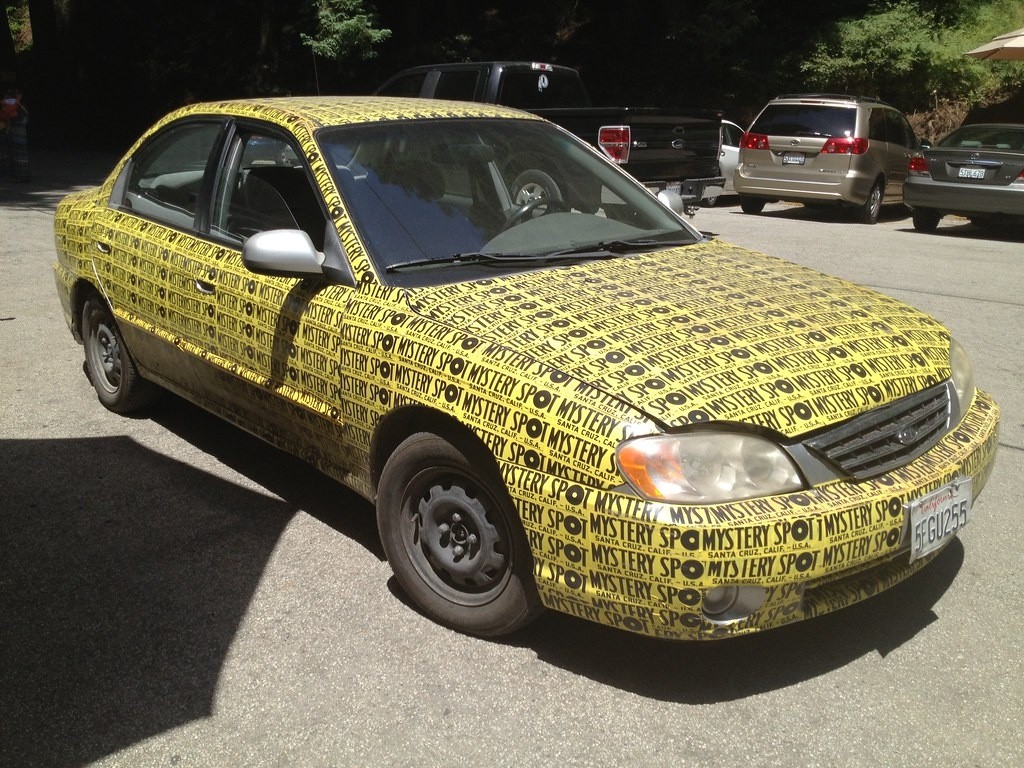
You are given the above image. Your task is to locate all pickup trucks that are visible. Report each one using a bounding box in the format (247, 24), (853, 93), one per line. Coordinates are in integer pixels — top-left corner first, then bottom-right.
(356, 60), (726, 222)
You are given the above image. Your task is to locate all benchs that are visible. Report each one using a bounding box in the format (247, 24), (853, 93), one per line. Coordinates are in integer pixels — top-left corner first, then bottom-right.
(153, 165), (354, 213)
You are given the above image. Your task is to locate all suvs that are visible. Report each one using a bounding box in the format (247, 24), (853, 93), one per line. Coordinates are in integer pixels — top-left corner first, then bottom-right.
(733, 93), (935, 223)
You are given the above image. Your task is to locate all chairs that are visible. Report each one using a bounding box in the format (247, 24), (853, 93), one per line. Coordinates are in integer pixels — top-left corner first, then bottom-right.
(230, 165), (355, 252)
(361, 158), (480, 264)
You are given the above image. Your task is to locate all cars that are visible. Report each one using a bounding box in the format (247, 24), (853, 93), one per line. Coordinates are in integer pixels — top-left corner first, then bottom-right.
(51, 96), (1002, 639)
(695, 119), (750, 208)
(900, 123), (1024, 230)
